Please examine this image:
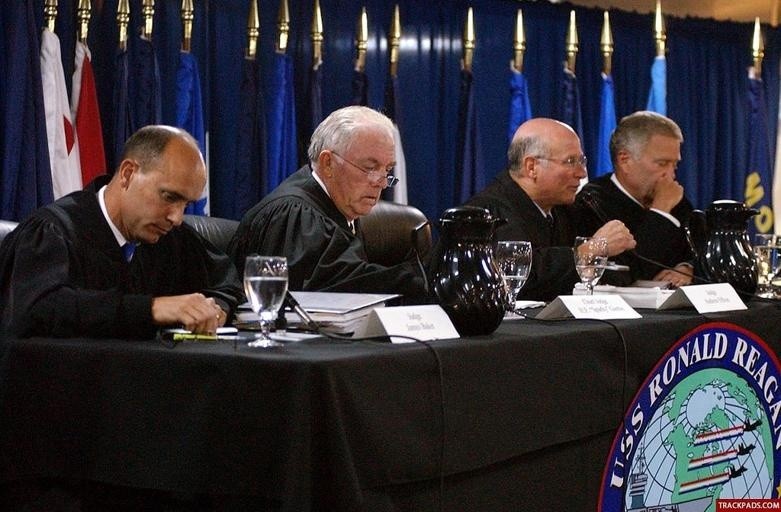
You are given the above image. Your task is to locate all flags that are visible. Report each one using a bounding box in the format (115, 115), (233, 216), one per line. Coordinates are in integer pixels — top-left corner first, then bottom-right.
(307, 64), (325, 132)
(265, 51), (299, 197)
(113, 43), (164, 174)
(349, 68), (370, 107)
(175, 52), (213, 217)
(646, 56), (669, 115)
(0, 0), (56, 222)
(237, 53), (265, 219)
(595, 71), (618, 177)
(451, 69), (486, 205)
(39, 28), (84, 200)
(70, 39), (108, 193)
(506, 66), (532, 146)
(557, 70), (586, 156)
(741, 78), (774, 247)
(382, 77), (413, 206)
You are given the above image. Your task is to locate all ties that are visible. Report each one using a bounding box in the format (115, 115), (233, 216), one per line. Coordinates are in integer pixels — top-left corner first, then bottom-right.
(124, 242), (137, 262)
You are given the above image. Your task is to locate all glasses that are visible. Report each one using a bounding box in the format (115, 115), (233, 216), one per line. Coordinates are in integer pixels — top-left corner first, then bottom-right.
(536, 155), (588, 167)
(330, 151), (399, 187)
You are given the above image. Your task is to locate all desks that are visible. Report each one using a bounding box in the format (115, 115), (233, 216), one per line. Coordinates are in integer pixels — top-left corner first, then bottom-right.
(8, 290), (781, 511)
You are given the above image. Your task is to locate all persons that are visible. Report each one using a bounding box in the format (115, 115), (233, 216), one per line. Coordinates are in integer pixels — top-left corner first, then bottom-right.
(429, 116), (638, 300)
(224, 105), (440, 306)
(0, 123), (244, 336)
(572, 109), (705, 288)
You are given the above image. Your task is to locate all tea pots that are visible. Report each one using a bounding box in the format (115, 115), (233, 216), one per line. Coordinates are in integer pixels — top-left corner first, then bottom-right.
(684, 201), (761, 306)
(411, 207), (509, 339)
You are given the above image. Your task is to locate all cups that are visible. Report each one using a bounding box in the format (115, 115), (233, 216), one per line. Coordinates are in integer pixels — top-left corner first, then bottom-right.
(243, 256), (288, 348)
(754, 233), (781, 276)
(573, 236), (608, 295)
(755, 245), (777, 297)
(494, 240), (533, 319)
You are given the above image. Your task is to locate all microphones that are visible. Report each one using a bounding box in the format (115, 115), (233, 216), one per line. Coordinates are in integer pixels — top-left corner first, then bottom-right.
(579, 192), (636, 258)
(247, 252), (318, 333)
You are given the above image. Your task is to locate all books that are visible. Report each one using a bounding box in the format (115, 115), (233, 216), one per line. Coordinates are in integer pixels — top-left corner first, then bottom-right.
(233, 289), (404, 336)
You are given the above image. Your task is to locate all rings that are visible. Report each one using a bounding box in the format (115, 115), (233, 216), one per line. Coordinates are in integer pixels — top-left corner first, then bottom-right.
(216, 314), (220, 319)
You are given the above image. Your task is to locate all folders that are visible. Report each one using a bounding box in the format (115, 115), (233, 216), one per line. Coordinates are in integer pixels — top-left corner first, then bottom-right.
(236, 288), (404, 315)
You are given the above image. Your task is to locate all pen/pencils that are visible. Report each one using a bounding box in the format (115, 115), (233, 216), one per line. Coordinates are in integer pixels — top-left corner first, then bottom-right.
(173, 332), (247, 341)
